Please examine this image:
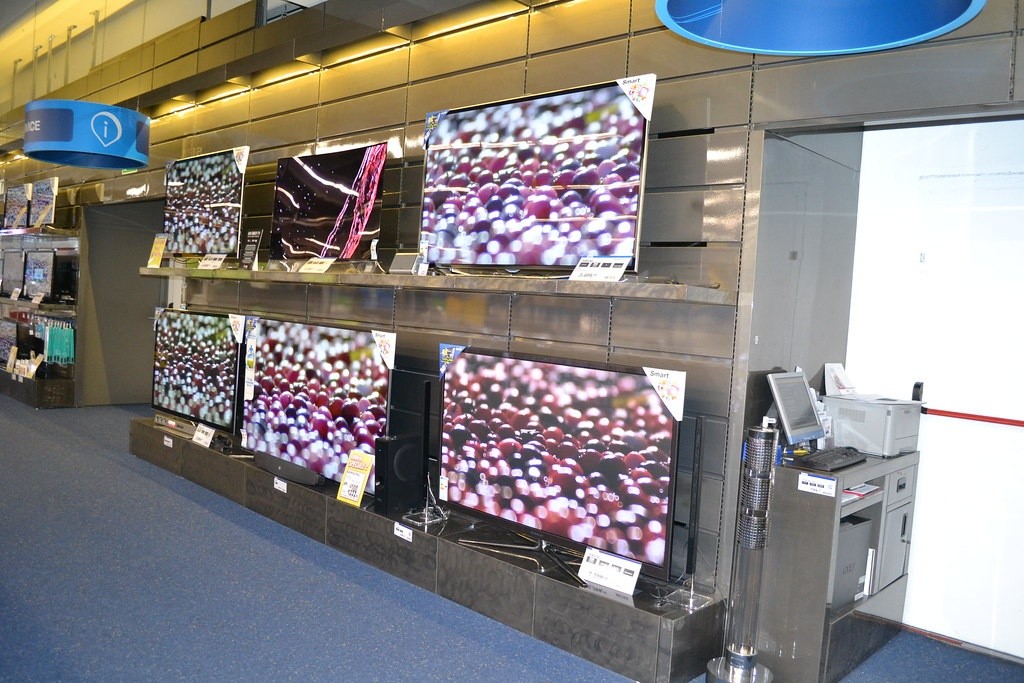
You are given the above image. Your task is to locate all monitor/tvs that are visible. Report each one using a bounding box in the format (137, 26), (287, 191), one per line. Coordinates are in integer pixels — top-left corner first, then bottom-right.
(766, 371), (824, 451)
(150, 73), (686, 582)
(0, 175), (59, 365)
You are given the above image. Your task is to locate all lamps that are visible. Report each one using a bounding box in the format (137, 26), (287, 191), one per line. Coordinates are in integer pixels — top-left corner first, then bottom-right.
(653, 0), (988, 57)
(24, 0), (150, 169)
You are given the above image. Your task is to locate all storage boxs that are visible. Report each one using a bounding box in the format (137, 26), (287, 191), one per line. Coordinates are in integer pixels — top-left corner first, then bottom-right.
(832, 514), (873, 610)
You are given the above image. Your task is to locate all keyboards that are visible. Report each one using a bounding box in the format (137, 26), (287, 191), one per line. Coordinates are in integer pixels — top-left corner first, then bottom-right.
(800, 446), (868, 471)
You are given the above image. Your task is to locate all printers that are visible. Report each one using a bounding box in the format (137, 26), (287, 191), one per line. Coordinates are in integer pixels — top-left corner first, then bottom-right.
(817, 363), (921, 457)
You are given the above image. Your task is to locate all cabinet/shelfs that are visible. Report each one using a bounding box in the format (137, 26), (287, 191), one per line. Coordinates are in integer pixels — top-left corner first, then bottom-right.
(0, 239), (80, 410)
(129, 267), (734, 683)
(725, 452), (920, 683)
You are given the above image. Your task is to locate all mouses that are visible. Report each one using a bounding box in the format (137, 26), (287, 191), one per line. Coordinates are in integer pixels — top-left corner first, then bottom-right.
(846, 446), (858, 452)
